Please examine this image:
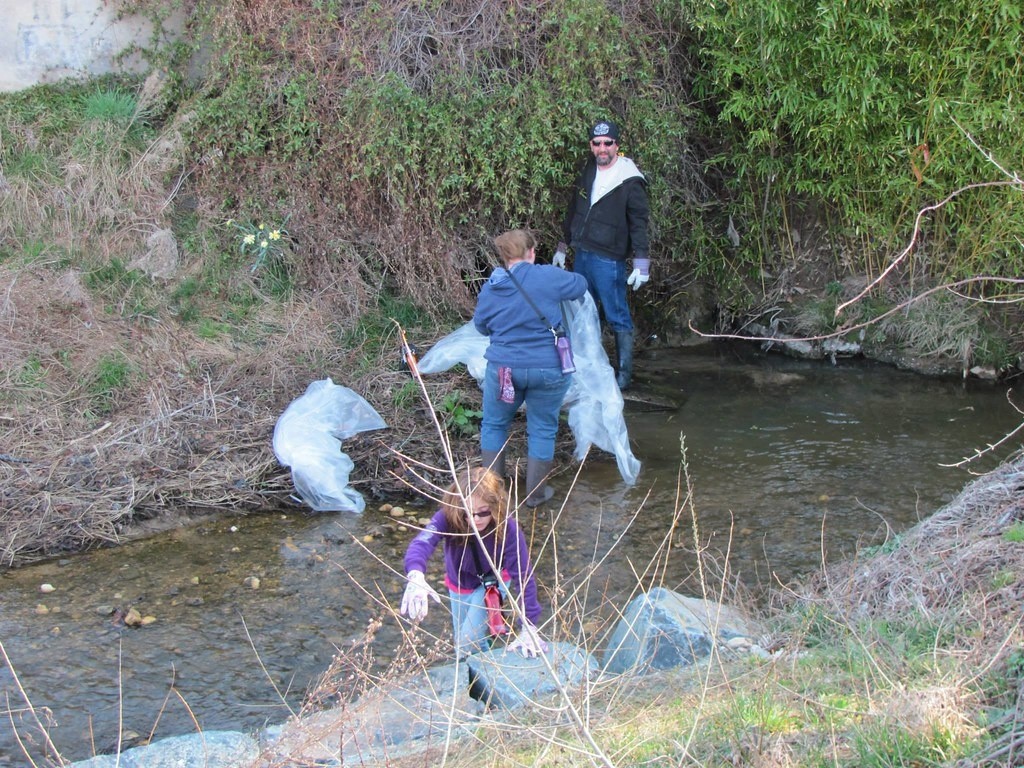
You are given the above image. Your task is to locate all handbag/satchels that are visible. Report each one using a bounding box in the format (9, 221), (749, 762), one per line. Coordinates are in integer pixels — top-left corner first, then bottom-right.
(486, 584), (506, 636)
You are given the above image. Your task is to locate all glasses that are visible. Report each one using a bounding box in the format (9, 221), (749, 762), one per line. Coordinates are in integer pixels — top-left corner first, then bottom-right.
(591, 140), (615, 146)
(463, 511), (492, 517)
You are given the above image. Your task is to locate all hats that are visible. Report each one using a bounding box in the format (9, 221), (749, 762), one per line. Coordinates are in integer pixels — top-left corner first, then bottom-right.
(589, 122), (618, 139)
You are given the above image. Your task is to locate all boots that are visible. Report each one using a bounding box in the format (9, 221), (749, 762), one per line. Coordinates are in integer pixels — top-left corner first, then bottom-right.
(525, 458), (554, 507)
(614, 328), (635, 390)
(481, 450), (506, 483)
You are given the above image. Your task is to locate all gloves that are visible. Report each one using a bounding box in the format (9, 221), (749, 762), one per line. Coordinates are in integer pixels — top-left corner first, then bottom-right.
(506, 622), (549, 657)
(401, 571), (440, 620)
(627, 259), (651, 292)
(552, 242), (571, 269)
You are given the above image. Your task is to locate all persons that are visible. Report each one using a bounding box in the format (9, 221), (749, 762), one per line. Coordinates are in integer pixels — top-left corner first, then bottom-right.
(552, 122), (650, 391)
(475, 229), (588, 505)
(400, 467), (549, 659)
